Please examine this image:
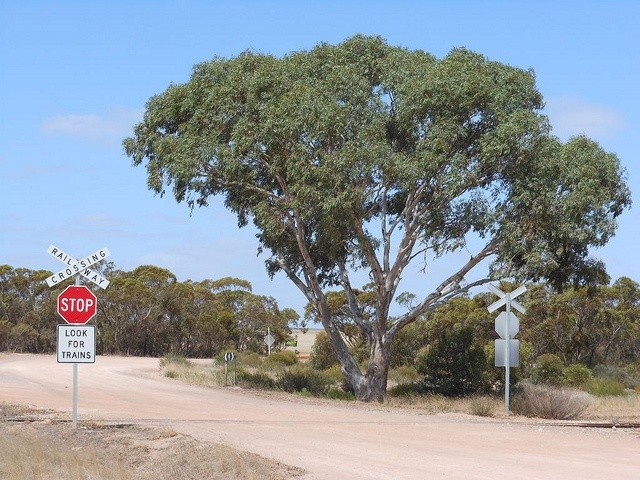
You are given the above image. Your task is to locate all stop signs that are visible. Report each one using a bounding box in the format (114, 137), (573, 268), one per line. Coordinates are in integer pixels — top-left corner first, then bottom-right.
(57, 285), (97, 325)
